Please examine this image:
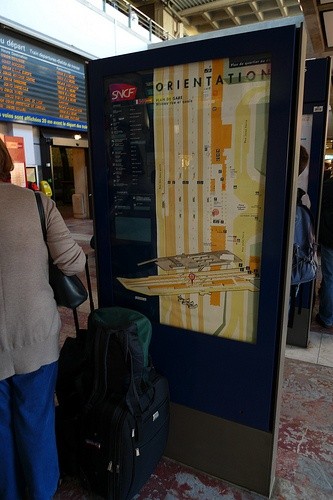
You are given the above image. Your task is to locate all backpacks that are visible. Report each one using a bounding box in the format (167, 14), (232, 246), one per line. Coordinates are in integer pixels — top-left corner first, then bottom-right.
(81, 306), (158, 416)
(291, 206), (317, 287)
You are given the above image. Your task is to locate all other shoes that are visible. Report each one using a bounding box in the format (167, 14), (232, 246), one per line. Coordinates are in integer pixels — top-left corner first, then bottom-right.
(316, 313), (332, 329)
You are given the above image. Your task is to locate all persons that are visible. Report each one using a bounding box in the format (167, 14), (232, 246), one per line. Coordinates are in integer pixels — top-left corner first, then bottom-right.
(0, 141), (86, 500)
(314, 161), (333, 330)
(296, 145), (310, 210)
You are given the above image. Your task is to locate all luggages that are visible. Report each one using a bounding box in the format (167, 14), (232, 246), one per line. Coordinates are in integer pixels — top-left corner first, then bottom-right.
(55, 252), (96, 417)
(78, 374), (169, 500)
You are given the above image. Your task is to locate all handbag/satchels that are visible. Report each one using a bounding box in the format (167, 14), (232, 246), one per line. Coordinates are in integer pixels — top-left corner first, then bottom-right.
(35, 191), (88, 309)
